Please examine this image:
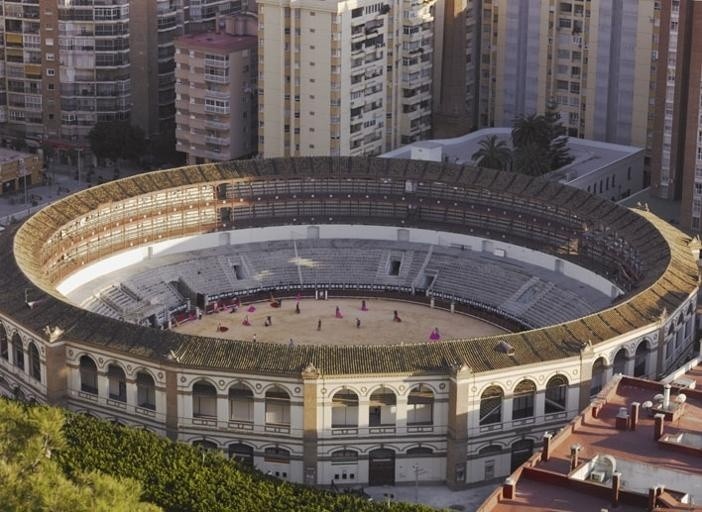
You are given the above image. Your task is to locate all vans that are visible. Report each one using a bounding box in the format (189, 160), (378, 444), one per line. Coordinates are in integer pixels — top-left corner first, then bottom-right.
(75, 169), (97, 182)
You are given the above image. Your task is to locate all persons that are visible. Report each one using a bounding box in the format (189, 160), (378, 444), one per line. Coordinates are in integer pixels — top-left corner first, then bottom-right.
(176, 289), (441, 346)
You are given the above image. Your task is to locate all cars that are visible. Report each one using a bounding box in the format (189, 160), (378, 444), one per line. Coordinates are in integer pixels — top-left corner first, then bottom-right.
(113, 168), (120, 177)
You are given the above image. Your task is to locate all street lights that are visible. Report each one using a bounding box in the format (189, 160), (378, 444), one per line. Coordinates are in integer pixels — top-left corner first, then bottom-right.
(412, 463), (420, 501)
(74, 147), (84, 187)
(381, 491), (394, 508)
(19, 158), (27, 209)
